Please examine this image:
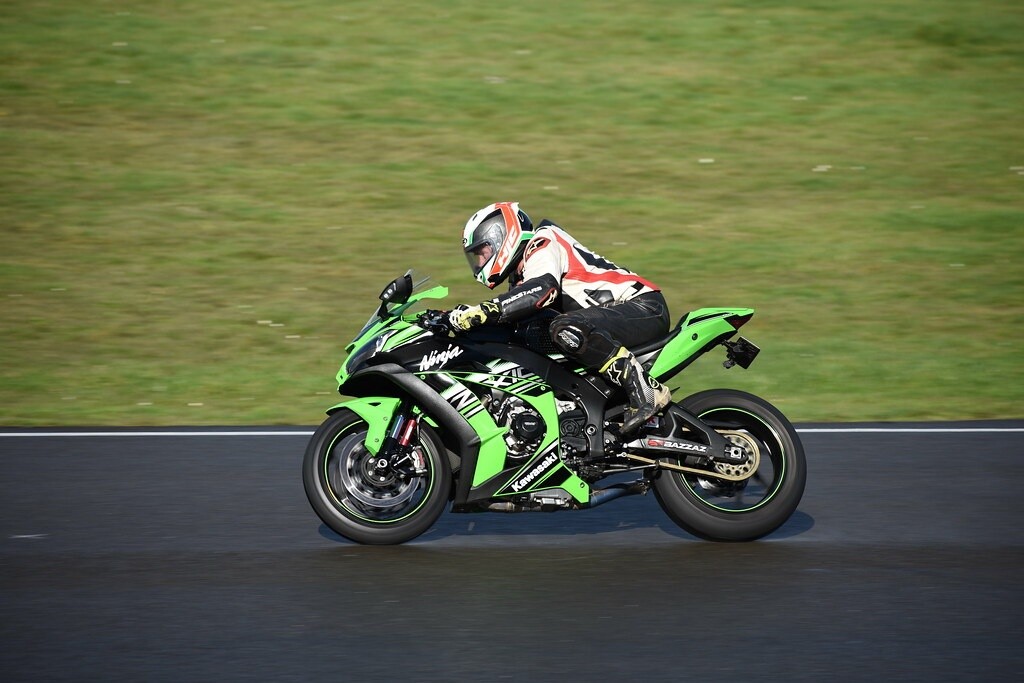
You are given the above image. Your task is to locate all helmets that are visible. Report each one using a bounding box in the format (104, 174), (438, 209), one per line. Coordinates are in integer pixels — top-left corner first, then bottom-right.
(460, 201), (535, 290)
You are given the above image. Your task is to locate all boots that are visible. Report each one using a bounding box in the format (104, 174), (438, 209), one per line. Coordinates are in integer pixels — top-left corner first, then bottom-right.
(598, 346), (672, 435)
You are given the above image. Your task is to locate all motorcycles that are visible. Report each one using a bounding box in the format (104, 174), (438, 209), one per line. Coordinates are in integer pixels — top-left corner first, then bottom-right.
(300, 267), (807, 545)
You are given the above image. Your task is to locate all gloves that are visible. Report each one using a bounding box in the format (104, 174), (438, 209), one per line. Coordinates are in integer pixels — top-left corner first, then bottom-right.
(435, 299), (503, 338)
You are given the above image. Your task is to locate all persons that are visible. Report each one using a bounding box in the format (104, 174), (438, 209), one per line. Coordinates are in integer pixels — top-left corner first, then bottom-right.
(447, 201), (673, 433)
